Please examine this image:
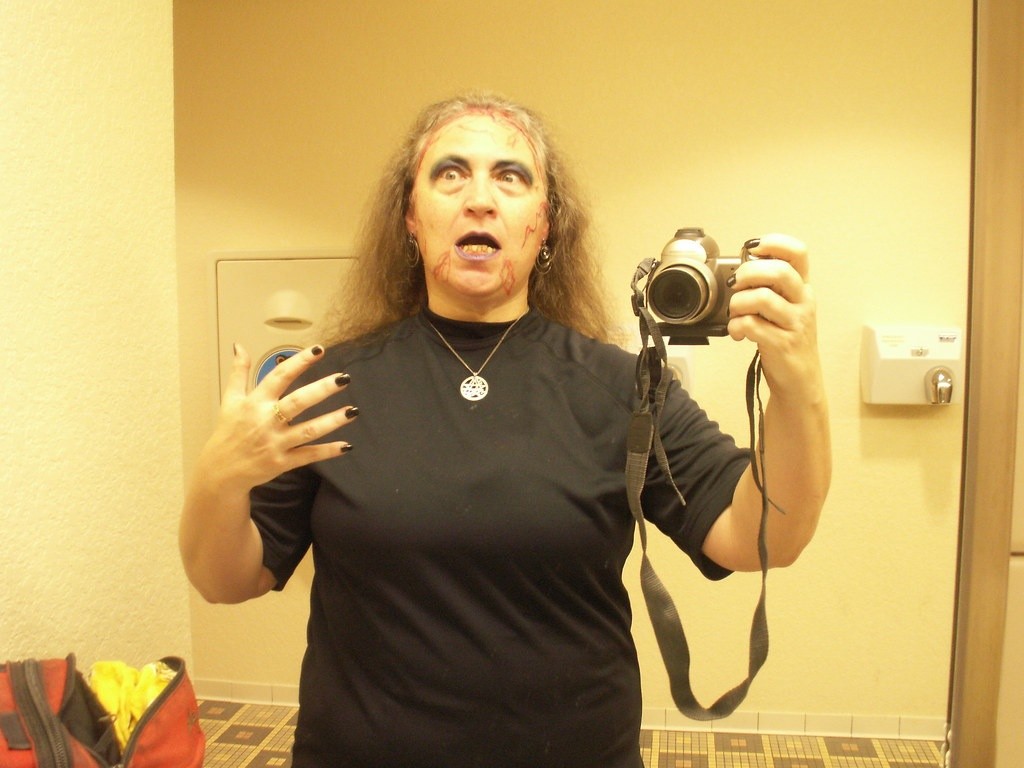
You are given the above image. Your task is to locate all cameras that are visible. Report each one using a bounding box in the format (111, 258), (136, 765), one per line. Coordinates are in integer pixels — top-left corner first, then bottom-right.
(630, 226), (773, 345)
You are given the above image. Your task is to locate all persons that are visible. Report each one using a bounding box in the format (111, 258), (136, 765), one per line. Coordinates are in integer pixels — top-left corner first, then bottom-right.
(178, 88), (832, 768)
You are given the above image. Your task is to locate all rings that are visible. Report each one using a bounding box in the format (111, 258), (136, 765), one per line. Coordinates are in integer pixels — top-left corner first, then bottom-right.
(273, 402), (293, 425)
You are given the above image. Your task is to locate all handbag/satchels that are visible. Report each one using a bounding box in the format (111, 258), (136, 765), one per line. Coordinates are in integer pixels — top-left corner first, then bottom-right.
(0, 653), (206, 768)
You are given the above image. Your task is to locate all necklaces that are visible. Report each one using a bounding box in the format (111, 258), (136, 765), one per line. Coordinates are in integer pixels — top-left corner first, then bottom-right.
(427, 303), (530, 402)
